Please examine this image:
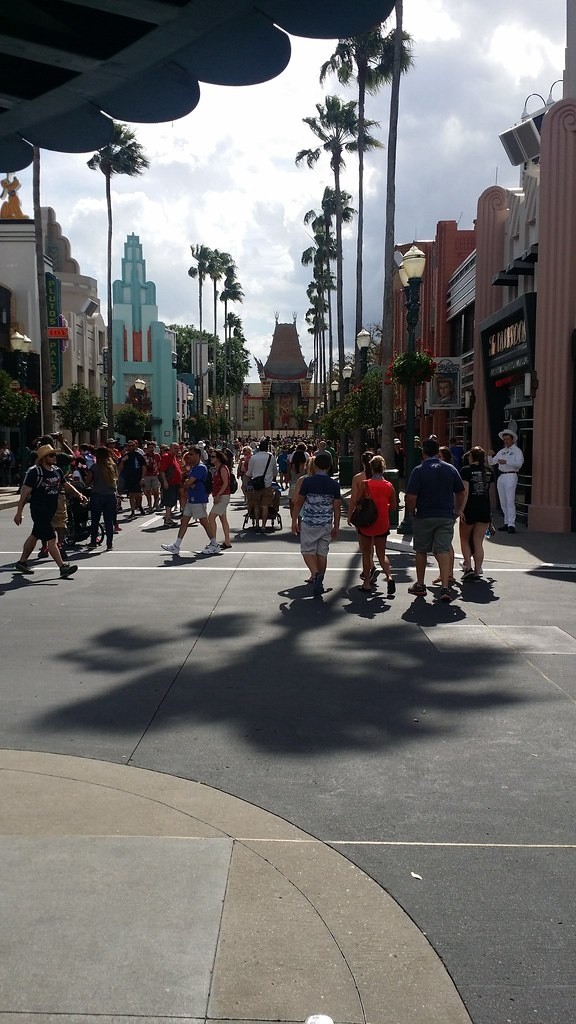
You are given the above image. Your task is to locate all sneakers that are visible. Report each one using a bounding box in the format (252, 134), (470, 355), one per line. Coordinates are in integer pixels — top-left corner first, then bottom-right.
(160, 544), (180, 554)
(202, 545), (221, 553)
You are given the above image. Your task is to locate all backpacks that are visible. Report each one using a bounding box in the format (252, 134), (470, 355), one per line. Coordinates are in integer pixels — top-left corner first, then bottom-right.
(197, 464), (213, 494)
(219, 464), (238, 494)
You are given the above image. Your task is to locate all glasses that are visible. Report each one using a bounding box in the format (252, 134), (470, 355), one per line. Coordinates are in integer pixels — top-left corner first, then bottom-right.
(47, 454), (56, 457)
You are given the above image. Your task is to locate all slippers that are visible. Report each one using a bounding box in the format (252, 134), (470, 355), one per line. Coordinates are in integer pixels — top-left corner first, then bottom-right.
(220, 543), (232, 548)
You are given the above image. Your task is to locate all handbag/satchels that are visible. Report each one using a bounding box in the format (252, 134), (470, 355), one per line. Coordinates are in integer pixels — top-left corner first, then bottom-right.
(251, 476), (265, 490)
(350, 480), (378, 526)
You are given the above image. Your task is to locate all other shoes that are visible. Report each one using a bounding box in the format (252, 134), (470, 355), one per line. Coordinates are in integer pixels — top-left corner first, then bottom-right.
(508, 526), (515, 533)
(256, 526), (265, 532)
(311, 573), (323, 596)
(498, 523), (508, 531)
(360, 565), (483, 601)
(16, 526), (122, 576)
(126, 507), (183, 527)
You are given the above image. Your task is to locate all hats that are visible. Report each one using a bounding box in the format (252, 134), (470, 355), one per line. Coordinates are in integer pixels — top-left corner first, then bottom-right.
(394, 439), (401, 444)
(429, 435), (437, 440)
(414, 436), (420, 441)
(105, 438), (118, 444)
(498, 429), (518, 442)
(36, 444), (61, 464)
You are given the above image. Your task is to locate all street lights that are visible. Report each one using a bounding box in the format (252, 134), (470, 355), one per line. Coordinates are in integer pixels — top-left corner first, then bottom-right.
(315, 402), (325, 442)
(396, 243), (426, 536)
(134, 379), (146, 448)
(331, 380), (339, 452)
(187, 392), (195, 442)
(356, 326), (372, 471)
(341, 364), (352, 455)
(224, 404), (229, 439)
(10, 331), (32, 492)
(206, 398), (213, 440)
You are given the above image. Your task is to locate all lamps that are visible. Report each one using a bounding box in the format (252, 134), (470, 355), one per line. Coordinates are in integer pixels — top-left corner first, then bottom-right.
(80, 297), (98, 318)
(522, 368), (539, 398)
(498, 79), (563, 164)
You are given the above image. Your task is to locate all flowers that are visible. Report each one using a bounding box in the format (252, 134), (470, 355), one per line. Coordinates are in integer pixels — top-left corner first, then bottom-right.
(382, 345), (438, 391)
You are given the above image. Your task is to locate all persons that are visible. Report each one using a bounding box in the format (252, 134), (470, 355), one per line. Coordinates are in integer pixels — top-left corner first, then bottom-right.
(14, 444), (88, 579)
(450, 439), (463, 469)
(161, 446), (221, 554)
(414, 436), (423, 467)
(0, 439), (14, 487)
(405, 439), (495, 601)
(30, 433), (76, 557)
(208, 450), (232, 548)
(70, 434), (354, 532)
(487, 429), (524, 534)
(394, 438), (405, 490)
(292, 455), (341, 594)
(86, 446), (117, 551)
(347, 451), (396, 594)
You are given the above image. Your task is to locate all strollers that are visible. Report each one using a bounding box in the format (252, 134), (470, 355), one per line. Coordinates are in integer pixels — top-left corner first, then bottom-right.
(242, 476), (282, 530)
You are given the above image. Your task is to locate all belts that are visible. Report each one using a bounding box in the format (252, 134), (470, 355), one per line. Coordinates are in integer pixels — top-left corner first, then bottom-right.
(502, 471), (516, 473)
